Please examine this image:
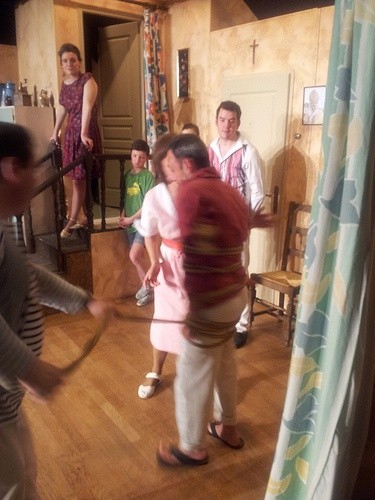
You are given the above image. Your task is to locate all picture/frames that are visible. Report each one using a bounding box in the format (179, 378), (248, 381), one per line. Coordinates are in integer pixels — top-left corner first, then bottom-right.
(177, 48), (189, 98)
(302, 85), (326, 126)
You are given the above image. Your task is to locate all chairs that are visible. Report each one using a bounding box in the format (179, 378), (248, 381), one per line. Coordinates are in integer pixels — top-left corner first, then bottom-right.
(246, 201), (313, 347)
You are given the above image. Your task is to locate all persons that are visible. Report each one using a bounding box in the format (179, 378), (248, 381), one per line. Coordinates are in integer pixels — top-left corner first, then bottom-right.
(49, 44), (104, 236)
(122, 101), (283, 467)
(0, 124), (117, 500)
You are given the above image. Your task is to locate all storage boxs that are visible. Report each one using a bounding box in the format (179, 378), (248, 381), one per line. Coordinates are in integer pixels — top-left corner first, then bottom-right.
(14, 94), (32, 106)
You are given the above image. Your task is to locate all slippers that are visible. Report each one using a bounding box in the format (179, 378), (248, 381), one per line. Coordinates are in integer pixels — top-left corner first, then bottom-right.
(207, 421), (245, 449)
(157, 444), (208, 467)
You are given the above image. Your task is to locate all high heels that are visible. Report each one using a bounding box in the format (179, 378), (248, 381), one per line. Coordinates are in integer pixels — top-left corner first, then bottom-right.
(70, 211), (93, 229)
(61, 217), (77, 239)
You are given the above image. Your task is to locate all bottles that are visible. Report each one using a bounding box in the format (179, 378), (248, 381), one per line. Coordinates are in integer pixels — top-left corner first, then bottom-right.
(40, 90), (46, 107)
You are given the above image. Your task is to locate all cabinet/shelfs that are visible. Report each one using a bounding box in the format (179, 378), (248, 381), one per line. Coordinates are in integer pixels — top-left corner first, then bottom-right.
(0, 106), (57, 236)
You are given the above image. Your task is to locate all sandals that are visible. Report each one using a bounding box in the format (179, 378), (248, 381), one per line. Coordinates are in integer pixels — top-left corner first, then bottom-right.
(137, 373), (163, 399)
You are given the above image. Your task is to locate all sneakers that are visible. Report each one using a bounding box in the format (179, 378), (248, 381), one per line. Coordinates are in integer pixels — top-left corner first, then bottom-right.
(135, 285), (152, 306)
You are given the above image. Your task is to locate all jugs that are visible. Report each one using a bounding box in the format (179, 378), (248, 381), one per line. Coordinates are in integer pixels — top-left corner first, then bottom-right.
(5, 83), (16, 105)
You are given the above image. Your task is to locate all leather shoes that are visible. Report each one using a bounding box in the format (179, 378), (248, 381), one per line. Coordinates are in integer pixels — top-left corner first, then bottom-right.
(234, 332), (247, 348)
